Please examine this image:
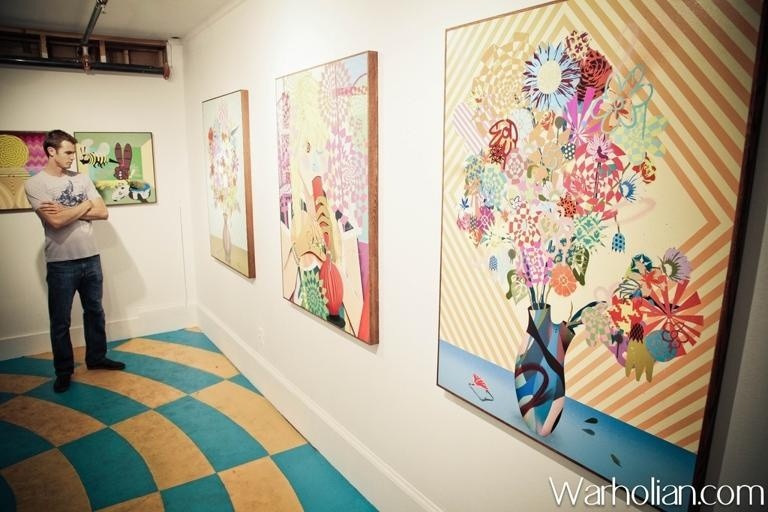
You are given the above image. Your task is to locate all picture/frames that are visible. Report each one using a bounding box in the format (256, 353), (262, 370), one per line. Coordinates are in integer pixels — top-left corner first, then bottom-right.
(273, 51), (380, 346)
(73, 131), (157, 210)
(200, 89), (257, 278)
(0, 130), (51, 212)
(435, 1), (766, 512)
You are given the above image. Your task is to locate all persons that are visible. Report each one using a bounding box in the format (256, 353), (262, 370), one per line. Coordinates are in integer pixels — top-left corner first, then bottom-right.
(24, 130), (126, 392)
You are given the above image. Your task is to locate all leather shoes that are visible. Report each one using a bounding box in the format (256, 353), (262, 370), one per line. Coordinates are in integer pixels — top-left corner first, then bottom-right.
(53, 369), (71, 393)
(86, 358), (125, 370)
